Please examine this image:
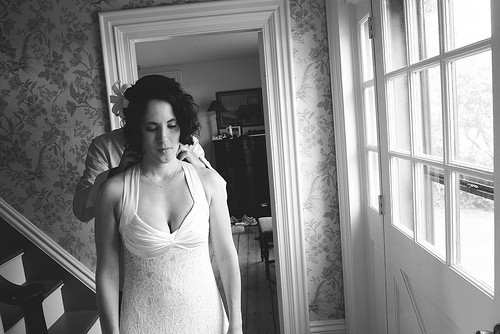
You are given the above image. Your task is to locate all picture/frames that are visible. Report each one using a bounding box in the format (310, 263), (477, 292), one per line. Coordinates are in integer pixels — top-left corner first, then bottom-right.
(138, 70), (182, 84)
(215, 88), (264, 129)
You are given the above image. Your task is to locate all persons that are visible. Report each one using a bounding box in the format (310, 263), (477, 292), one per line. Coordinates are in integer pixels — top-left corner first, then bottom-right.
(95, 75), (241, 334)
(73, 75), (247, 334)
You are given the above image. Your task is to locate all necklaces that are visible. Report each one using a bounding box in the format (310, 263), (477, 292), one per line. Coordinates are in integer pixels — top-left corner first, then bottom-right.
(141, 161), (179, 188)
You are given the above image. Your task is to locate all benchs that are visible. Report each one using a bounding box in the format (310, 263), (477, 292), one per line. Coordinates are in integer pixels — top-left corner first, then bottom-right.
(258, 216), (275, 279)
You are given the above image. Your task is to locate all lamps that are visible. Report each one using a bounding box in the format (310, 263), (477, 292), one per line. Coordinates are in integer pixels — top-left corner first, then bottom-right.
(207, 100), (227, 136)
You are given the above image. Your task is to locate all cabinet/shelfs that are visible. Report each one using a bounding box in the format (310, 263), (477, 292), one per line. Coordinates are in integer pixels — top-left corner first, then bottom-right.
(212, 136), (271, 221)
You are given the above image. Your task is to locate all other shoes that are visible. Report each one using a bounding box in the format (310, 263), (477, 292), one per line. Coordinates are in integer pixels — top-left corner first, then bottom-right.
(230, 215), (257, 226)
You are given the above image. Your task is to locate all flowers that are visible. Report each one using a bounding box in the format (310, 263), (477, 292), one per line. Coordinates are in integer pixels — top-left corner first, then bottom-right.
(110, 79), (132, 118)
